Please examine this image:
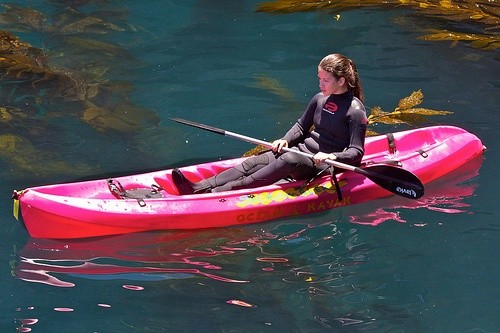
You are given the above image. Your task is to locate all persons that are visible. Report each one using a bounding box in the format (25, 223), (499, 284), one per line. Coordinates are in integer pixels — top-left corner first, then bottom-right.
(171, 53), (368, 195)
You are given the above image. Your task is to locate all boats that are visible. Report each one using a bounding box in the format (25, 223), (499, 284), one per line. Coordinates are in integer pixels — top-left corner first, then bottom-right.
(10, 125), (487, 240)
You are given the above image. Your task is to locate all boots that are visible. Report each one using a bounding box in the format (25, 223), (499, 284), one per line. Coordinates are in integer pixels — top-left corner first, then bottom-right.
(170, 169), (210, 193)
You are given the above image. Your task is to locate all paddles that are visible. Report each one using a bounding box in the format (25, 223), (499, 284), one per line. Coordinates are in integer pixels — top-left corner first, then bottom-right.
(172, 117), (424, 200)
